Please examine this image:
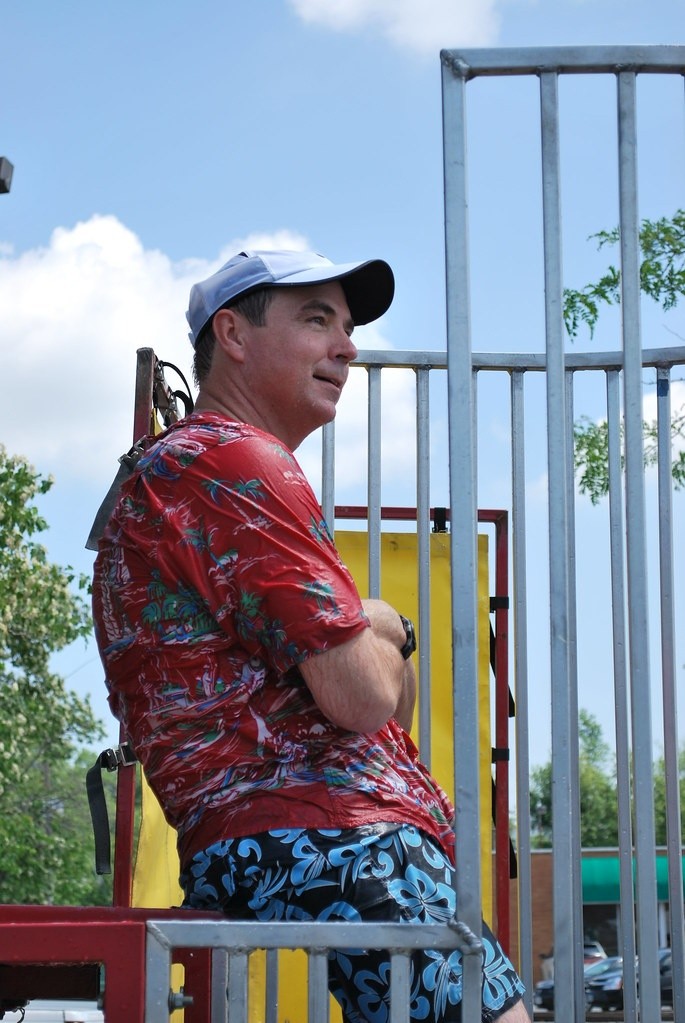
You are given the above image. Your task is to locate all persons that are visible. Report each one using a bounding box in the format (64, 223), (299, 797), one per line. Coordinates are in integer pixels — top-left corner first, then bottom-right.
(91, 248), (533, 1023)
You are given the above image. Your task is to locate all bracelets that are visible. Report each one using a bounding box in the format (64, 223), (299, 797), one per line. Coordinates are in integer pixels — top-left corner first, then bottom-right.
(399, 615), (417, 661)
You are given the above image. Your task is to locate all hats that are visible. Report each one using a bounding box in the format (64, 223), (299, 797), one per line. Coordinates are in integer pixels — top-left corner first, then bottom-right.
(187, 247), (396, 350)
